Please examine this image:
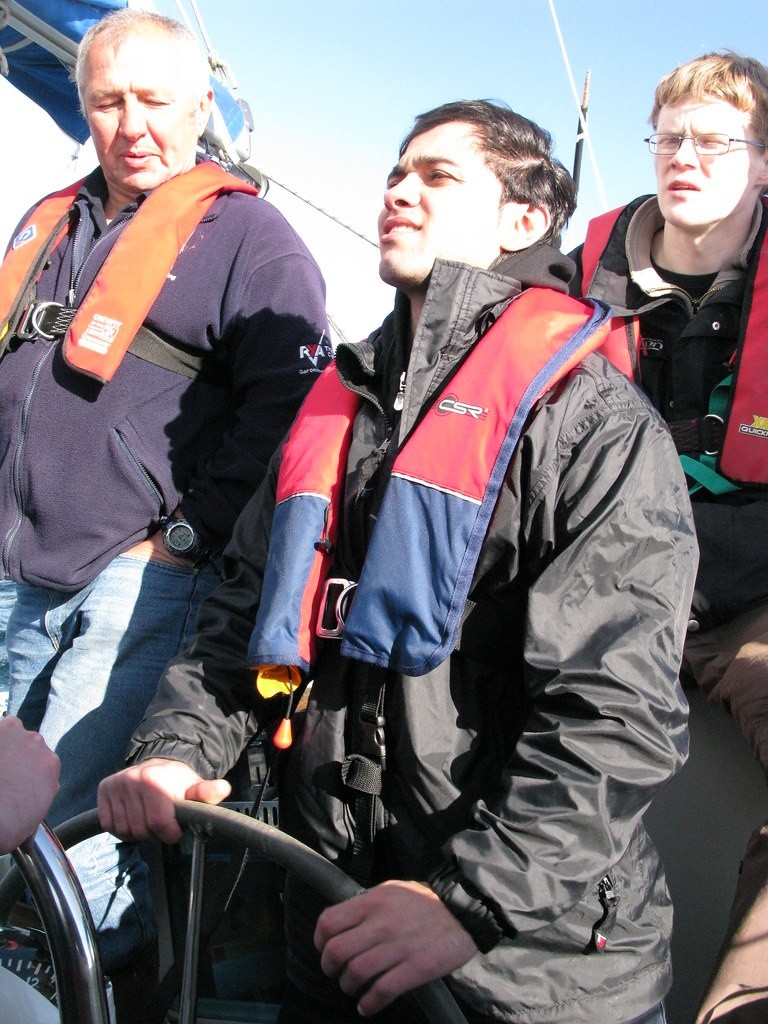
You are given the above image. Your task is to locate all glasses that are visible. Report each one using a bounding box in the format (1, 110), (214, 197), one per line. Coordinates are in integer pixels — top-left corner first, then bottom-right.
(644, 132), (766, 155)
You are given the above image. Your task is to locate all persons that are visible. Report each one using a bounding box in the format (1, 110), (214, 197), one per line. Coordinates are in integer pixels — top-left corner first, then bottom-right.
(0, 6), (334, 1024)
(98, 98), (699, 1024)
(564, 52), (768, 1024)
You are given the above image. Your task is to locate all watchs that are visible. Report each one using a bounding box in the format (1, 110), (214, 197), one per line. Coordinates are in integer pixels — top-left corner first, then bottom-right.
(162, 516), (207, 561)
